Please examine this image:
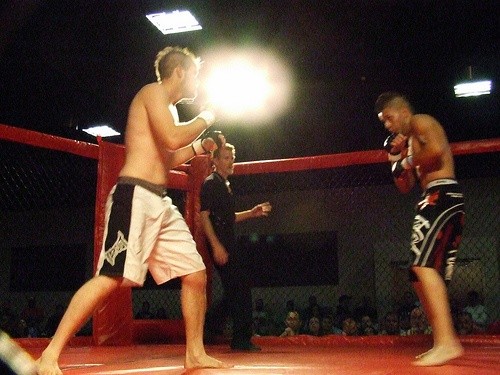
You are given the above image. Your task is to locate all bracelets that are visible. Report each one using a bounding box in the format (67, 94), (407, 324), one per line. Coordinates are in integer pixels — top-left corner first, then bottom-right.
(193, 139), (205, 155)
(401, 155), (413, 170)
(197, 111), (215, 129)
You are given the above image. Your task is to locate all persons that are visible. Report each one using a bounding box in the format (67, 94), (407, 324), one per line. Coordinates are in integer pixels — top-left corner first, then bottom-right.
(199, 143), (271, 350)
(0, 296), (68, 338)
(135, 302), (167, 319)
(252, 293), (433, 335)
(374, 92), (466, 367)
(35, 47), (235, 375)
(446, 286), (488, 334)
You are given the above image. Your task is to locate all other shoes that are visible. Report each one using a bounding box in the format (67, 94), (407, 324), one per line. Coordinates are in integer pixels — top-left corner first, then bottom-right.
(230, 341), (262, 352)
